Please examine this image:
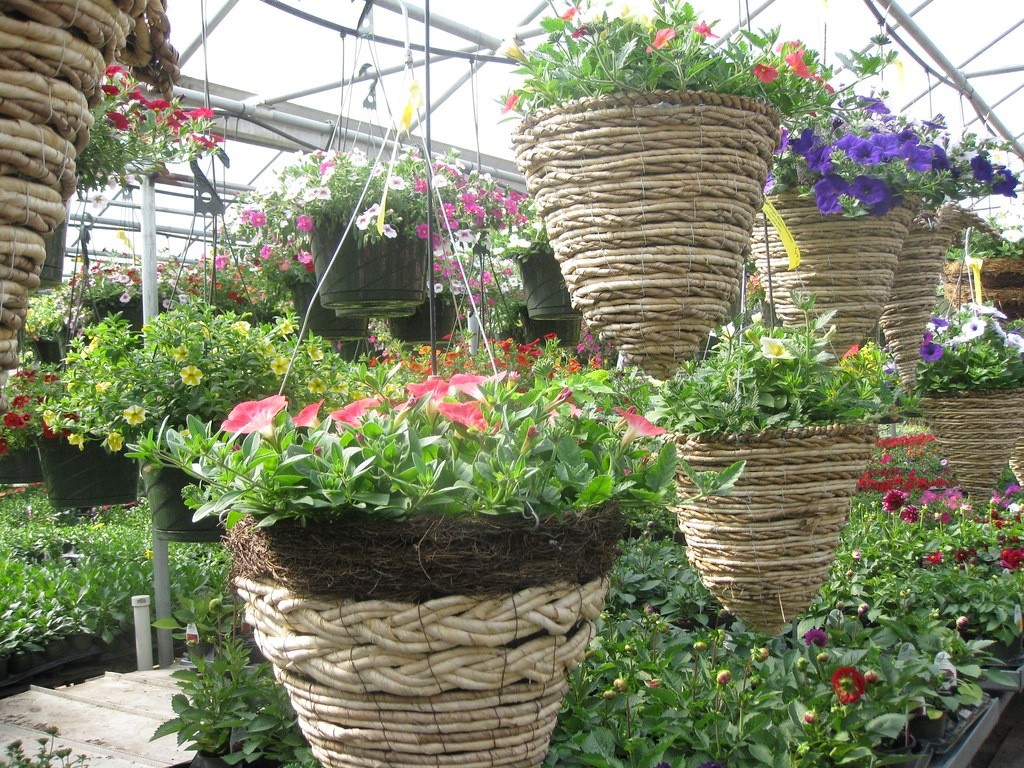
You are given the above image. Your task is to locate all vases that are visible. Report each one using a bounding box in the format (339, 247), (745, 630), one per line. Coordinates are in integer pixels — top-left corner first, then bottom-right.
(910, 711), (946, 739)
(986, 634), (1019, 661)
(139, 452), (234, 543)
(257, 312), (291, 341)
(29, 330), (91, 372)
(519, 306), (581, 347)
(309, 216), (428, 310)
(335, 307), (416, 318)
(230, 569), (611, 768)
(508, 90), (782, 381)
(388, 295), (457, 344)
(1008, 431), (1024, 487)
(877, 208), (964, 394)
(500, 327), (525, 346)
(656, 422), (880, 638)
(956, 630), (976, 653)
(0, 0), (183, 420)
(33, 431), (139, 507)
(199, 747), (232, 768)
(875, 733), (916, 754)
(750, 190), (922, 367)
(519, 248), (583, 320)
(93, 291), (172, 349)
(344, 337), (390, 368)
(915, 386), (1024, 507)
(291, 282), (370, 340)
(0, 445), (45, 484)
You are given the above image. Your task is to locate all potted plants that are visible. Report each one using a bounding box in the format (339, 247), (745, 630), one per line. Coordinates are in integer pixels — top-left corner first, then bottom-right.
(152, 559), (229, 662)
(1, 524), (186, 680)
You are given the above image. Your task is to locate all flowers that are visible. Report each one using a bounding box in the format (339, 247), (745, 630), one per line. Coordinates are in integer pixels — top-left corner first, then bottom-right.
(776, 630), (967, 767)
(0, 0), (1024, 625)
(149, 598), (276, 755)
